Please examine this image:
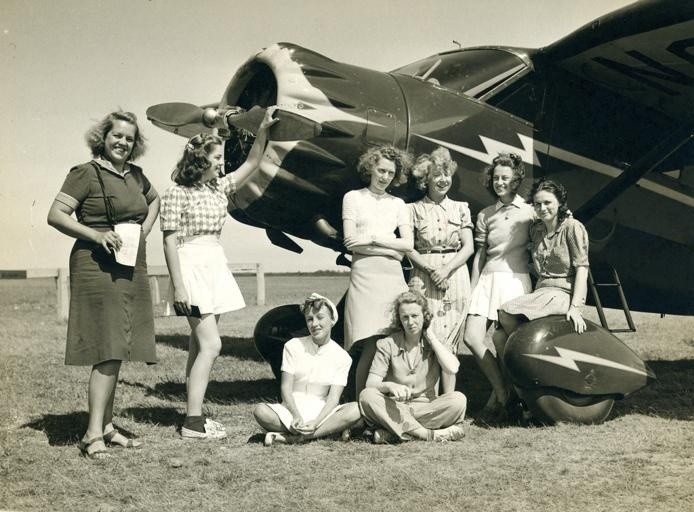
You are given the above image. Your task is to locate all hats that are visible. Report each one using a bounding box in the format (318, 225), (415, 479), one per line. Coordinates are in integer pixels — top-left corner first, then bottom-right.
(304, 292), (340, 327)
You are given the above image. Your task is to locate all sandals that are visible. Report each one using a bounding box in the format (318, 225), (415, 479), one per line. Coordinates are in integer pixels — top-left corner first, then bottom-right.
(76, 436), (114, 459)
(104, 429), (146, 451)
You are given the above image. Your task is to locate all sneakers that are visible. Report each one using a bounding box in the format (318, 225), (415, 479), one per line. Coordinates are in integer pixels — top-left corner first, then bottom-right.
(372, 428), (395, 444)
(434, 426), (466, 443)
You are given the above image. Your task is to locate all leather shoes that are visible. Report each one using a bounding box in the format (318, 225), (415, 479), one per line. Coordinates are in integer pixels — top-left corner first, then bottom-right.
(181, 423), (226, 439)
(473, 405), (497, 426)
(491, 387), (520, 423)
(205, 418), (225, 431)
(265, 430), (286, 446)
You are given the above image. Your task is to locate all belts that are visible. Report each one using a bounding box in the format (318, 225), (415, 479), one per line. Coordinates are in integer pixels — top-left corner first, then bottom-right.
(417, 248), (460, 255)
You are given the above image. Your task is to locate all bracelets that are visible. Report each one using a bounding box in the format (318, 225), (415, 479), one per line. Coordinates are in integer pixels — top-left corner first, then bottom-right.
(569, 302), (585, 311)
(367, 234), (375, 245)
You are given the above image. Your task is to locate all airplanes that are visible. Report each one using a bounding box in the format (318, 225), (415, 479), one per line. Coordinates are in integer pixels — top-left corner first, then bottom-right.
(145, 0), (693, 428)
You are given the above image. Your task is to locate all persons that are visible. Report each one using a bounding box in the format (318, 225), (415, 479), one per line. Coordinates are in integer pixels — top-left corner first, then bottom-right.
(341, 144), (415, 400)
(358, 292), (471, 446)
(160, 106), (279, 436)
(402, 147), (475, 394)
(253, 292), (364, 448)
(492, 177), (591, 424)
(463, 152), (573, 425)
(47, 108), (160, 462)
